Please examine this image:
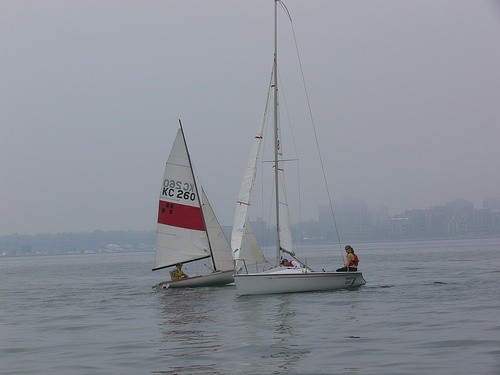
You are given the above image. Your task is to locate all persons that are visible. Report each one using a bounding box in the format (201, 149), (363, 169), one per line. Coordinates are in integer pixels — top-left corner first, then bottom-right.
(281, 258), (300, 269)
(336, 245), (360, 273)
(169, 263), (189, 281)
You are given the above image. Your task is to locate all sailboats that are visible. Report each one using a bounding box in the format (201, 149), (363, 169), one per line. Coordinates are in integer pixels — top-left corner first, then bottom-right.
(232, 0), (367, 296)
(151, 118), (243, 290)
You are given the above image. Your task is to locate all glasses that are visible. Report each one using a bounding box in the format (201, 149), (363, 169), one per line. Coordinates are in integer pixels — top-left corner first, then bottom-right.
(345, 248), (350, 250)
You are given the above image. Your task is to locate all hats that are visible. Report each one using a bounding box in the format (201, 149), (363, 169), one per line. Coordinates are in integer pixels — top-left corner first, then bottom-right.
(176, 263), (183, 267)
